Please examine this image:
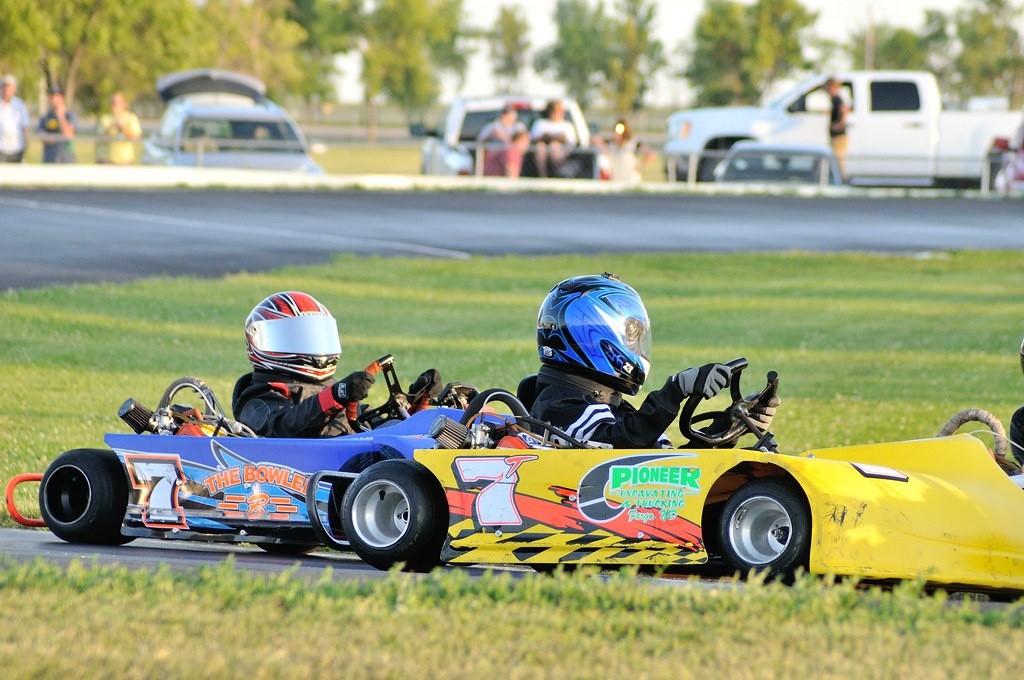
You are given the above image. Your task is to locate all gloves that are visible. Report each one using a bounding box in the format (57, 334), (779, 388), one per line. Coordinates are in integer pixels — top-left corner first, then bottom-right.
(711, 391), (781, 446)
(404, 368), (444, 407)
(672, 363), (732, 400)
(331, 370), (375, 408)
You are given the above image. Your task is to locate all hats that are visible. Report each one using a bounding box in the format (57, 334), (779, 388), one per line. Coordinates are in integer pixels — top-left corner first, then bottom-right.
(504, 102), (518, 112)
(1, 75), (17, 84)
(47, 86), (62, 94)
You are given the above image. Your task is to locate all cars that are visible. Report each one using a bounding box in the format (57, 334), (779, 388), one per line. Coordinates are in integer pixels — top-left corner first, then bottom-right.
(144, 69), (322, 178)
(714, 139), (842, 192)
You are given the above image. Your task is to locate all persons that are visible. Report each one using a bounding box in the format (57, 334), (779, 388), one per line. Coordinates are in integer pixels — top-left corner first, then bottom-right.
(825, 76), (851, 183)
(592, 117), (653, 185)
(471, 99), (579, 178)
(0, 72), (148, 166)
(527, 270), (781, 447)
(233, 288), (445, 440)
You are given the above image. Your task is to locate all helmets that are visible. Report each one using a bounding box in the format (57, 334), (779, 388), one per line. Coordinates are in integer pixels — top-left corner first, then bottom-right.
(245, 292), (342, 383)
(535, 272), (652, 397)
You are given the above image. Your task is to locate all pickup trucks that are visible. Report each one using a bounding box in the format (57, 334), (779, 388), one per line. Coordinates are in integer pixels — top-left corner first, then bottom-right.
(408, 97), (600, 183)
(664, 68), (1024, 190)
